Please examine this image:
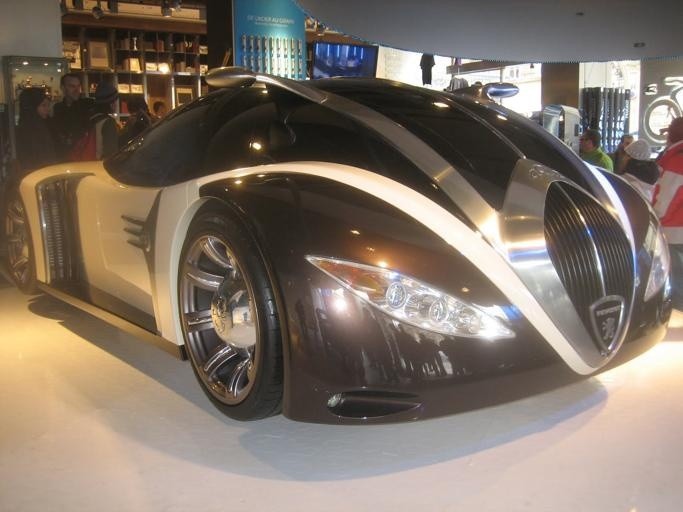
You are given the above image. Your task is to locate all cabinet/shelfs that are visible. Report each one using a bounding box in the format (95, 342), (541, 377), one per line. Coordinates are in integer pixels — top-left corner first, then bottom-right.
(61, 12), (211, 123)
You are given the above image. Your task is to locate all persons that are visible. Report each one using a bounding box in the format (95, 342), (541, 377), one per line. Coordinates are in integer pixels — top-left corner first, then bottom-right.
(18, 73), (165, 163)
(580, 117), (683, 309)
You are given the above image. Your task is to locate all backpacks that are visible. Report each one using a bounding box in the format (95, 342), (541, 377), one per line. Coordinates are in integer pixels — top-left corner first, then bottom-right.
(70, 113), (110, 160)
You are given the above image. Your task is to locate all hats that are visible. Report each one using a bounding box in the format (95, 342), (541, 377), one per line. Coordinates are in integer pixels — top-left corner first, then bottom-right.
(624, 139), (651, 161)
(94, 82), (119, 106)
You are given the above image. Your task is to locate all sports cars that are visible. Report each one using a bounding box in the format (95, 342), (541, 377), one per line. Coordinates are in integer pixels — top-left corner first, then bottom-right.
(0, 66), (673, 425)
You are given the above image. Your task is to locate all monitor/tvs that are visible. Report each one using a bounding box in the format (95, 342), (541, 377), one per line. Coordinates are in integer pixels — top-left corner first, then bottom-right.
(310, 40), (379, 80)
